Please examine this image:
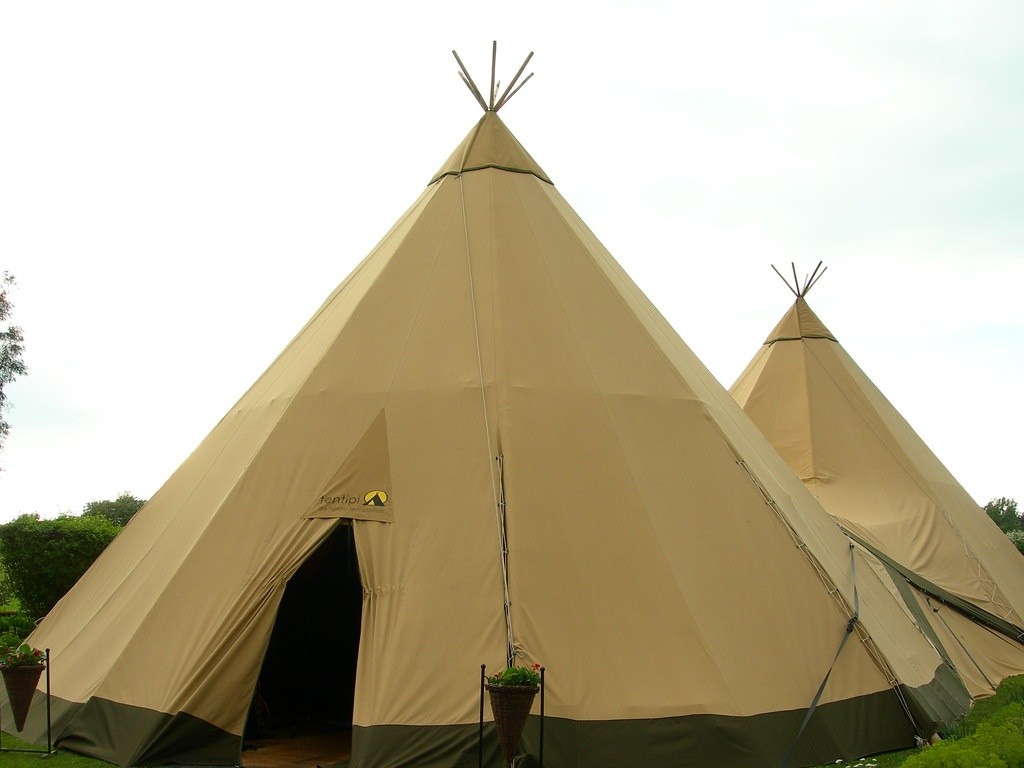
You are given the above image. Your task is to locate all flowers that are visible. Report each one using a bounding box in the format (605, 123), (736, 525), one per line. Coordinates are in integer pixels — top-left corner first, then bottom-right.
(0, 642), (47, 668)
(485, 662), (542, 687)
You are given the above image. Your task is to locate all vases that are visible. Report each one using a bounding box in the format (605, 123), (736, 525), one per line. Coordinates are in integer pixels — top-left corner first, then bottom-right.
(487, 684), (539, 767)
(0, 664), (46, 732)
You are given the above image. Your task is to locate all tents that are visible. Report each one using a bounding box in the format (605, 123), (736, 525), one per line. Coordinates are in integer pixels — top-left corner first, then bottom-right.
(729, 263), (1024, 699)
(0, 45), (976, 768)
